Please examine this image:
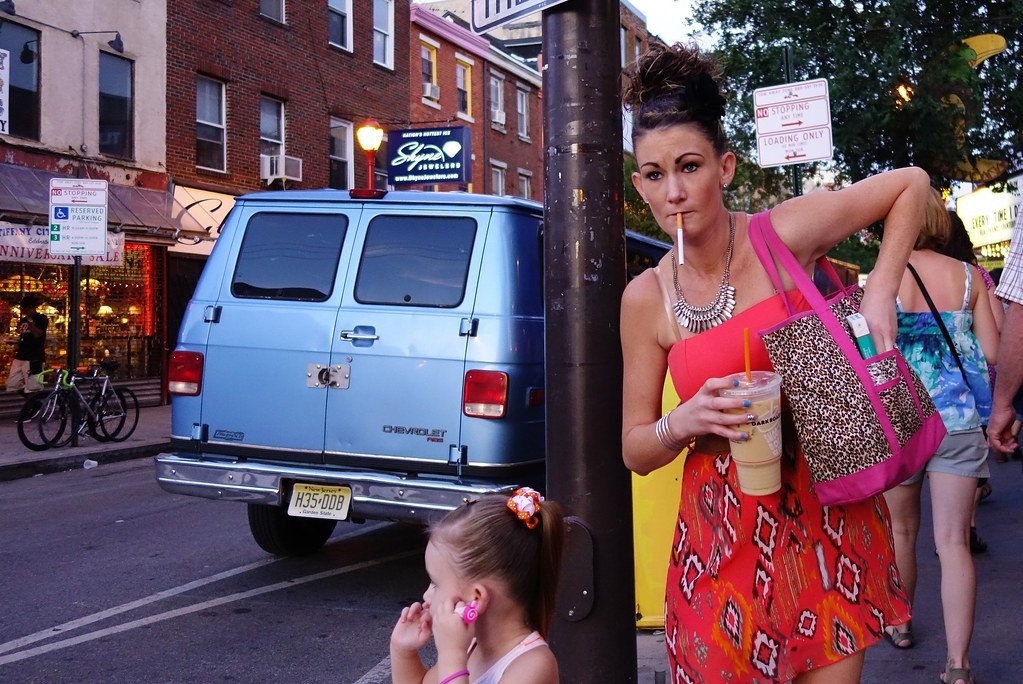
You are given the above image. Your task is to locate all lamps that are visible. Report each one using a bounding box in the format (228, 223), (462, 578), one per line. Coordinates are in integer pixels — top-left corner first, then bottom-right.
(21, 39), (39, 65)
(491, 110), (506, 125)
(113, 223), (218, 244)
(70, 29), (124, 54)
(422, 83), (440, 100)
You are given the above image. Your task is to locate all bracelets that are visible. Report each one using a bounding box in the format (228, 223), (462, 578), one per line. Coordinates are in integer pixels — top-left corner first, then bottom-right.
(655, 410), (693, 452)
(439, 670), (470, 684)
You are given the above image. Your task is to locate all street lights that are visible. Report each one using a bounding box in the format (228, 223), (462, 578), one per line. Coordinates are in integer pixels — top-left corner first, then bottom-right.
(355, 118), (384, 190)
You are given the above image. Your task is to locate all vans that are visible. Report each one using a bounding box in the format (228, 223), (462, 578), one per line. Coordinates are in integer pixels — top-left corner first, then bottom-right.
(155, 185), (674, 562)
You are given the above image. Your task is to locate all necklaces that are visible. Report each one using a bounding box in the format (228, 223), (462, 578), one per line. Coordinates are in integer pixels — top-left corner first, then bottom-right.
(671, 211), (736, 334)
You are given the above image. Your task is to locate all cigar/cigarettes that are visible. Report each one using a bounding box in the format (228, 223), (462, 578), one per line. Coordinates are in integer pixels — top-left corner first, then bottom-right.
(677, 212), (684, 265)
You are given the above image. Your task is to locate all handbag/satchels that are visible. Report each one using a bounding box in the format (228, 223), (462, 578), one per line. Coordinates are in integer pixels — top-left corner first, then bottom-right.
(748, 209), (948, 507)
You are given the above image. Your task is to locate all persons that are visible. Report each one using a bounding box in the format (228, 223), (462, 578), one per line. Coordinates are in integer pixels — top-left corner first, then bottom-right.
(5, 294), (49, 423)
(618, 47), (929, 684)
(49, 311), (61, 334)
(987, 164), (1022, 453)
(82, 313), (142, 355)
(857, 181), (1006, 683)
(10, 311), (19, 333)
(390, 484), (565, 684)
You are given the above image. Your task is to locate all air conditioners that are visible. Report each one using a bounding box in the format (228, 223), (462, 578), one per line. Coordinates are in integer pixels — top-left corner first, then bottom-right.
(260, 154), (302, 182)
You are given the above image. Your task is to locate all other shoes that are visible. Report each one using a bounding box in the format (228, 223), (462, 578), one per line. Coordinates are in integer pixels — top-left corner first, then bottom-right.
(934, 527), (986, 555)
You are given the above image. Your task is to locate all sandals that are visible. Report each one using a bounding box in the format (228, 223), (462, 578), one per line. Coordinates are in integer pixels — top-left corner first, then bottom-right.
(885, 626), (914, 650)
(940, 657), (974, 684)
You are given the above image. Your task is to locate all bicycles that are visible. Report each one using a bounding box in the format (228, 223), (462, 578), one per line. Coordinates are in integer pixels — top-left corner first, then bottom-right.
(17, 367), (139, 451)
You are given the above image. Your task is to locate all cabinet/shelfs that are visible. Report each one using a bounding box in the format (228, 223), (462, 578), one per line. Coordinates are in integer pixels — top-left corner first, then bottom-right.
(79, 335), (153, 376)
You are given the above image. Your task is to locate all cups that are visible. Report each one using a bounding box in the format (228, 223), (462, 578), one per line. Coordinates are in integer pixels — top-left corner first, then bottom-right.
(84, 460), (98, 469)
(718, 371), (782, 496)
